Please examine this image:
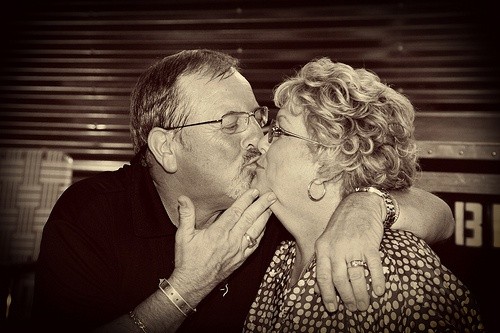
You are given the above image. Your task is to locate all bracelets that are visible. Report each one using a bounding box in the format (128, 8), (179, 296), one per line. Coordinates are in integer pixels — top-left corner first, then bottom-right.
(342, 183), (400, 229)
(127, 311), (151, 333)
(158, 278), (197, 317)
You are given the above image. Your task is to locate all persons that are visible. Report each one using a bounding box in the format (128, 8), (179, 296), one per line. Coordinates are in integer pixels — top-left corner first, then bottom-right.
(243, 56), (486, 333)
(34, 49), (456, 333)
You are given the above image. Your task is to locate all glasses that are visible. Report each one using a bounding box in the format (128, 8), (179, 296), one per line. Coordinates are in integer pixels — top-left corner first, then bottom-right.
(164, 106), (268, 134)
(268, 119), (334, 148)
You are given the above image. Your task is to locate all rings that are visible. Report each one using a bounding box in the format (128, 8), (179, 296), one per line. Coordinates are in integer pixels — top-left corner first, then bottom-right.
(347, 259), (366, 269)
(243, 232), (258, 248)
